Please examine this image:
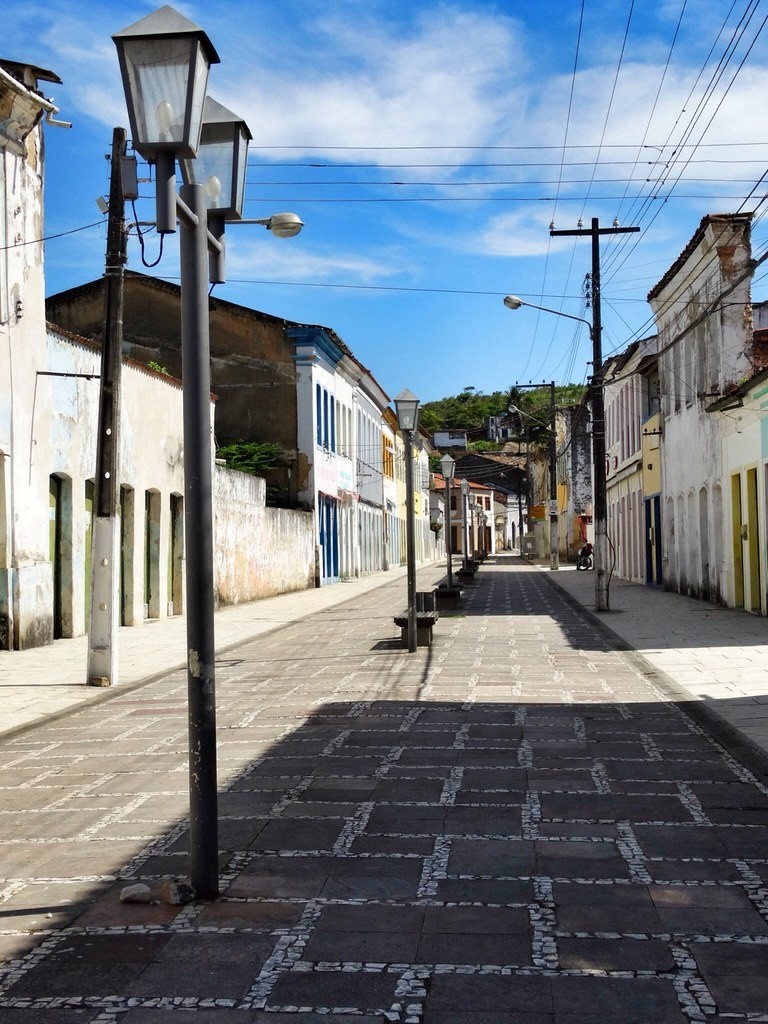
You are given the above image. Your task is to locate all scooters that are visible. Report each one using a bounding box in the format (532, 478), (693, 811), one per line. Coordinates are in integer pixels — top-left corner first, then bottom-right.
(575, 539), (595, 570)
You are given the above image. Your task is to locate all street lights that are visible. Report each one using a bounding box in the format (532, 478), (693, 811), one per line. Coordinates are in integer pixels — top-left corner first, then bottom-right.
(459, 477), (469, 571)
(438, 453), (458, 593)
(393, 387), (422, 653)
(468, 491), (489, 562)
(500, 294), (612, 612)
(84, 212), (305, 688)
(109, 6), (254, 899)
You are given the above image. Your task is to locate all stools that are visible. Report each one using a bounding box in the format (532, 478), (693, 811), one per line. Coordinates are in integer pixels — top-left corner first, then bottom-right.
(435, 588), (460, 611)
(439, 583), (463, 600)
(455, 571), (473, 584)
(393, 611), (440, 646)
(460, 568), (477, 581)
